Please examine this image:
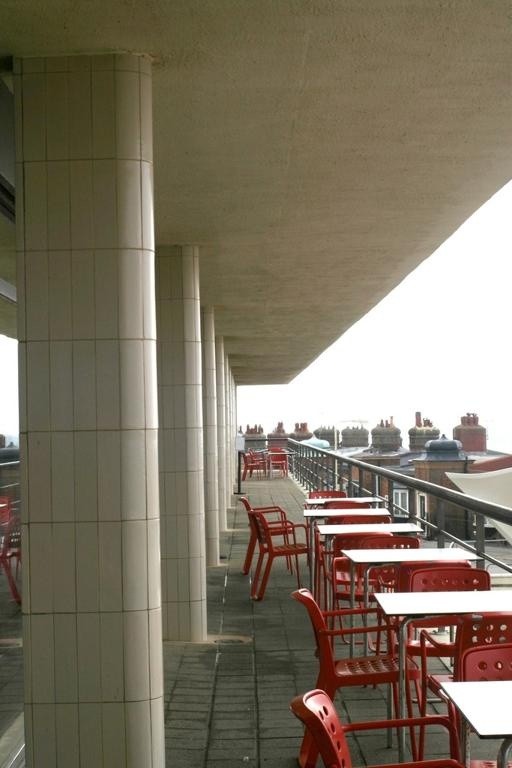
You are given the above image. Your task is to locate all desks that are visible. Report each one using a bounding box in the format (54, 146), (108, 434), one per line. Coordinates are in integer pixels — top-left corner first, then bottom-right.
(373, 587), (511, 676)
(438, 679), (512, 765)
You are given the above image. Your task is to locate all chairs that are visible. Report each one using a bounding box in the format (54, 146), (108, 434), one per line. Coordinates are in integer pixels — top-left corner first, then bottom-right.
(0, 516), (23, 609)
(238, 486), (511, 588)
(293, 586), (428, 764)
(239, 444), (296, 479)
(294, 686), (461, 766)
